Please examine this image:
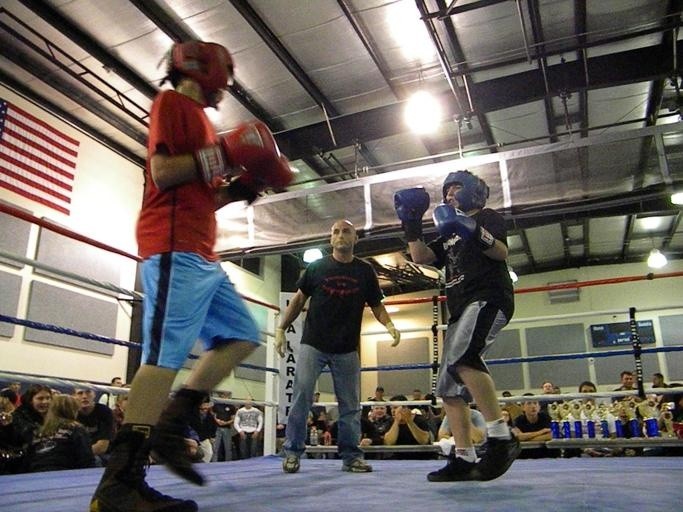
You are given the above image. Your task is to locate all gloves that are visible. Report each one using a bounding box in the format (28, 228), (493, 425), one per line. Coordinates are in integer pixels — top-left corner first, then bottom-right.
(221, 121), (281, 166)
(385, 322), (399, 347)
(393, 186), (429, 220)
(238, 151), (294, 192)
(273, 328), (286, 358)
(432, 205), (476, 240)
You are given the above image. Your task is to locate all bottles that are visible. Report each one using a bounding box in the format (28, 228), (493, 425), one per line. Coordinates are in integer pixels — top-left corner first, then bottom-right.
(309, 426), (318, 446)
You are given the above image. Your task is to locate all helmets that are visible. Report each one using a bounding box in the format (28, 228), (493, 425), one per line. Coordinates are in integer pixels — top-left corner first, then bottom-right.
(442, 171), (489, 211)
(168, 41), (234, 94)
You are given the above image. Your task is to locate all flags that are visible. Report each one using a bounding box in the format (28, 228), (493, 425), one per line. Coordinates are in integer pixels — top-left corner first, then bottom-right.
(1, 98), (80, 217)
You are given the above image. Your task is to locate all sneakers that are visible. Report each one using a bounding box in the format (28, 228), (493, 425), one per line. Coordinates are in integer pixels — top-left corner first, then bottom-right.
(427, 458), (475, 481)
(150, 427), (204, 486)
(341, 459), (372, 473)
(283, 455), (299, 472)
(471, 434), (521, 480)
(89, 466), (197, 512)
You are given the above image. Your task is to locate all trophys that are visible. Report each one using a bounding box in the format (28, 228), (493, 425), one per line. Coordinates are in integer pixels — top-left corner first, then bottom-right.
(608, 400), (627, 440)
(623, 398), (644, 440)
(596, 402), (612, 440)
(581, 401), (597, 439)
(570, 400), (584, 439)
(661, 402), (678, 439)
(636, 393), (663, 438)
(559, 401), (572, 439)
(547, 402), (562, 441)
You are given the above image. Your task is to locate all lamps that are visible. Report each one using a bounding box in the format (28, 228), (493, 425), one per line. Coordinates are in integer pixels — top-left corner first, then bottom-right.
(303, 248), (323, 263)
(506, 265), (518, 283)
(646, 248), (669, 270)
(671, 192), (683, 206)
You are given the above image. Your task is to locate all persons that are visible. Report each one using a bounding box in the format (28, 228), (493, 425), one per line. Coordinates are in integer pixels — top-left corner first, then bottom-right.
(366, 405), (392, 434)
(197, 402), (214, 463)
(99, 378), (122, 411)
(408, 390), (424, 410)
(512, 393), (552, 441)
(0, 390), (18, 414)
(235, 396), (263, 458)
(111, 385), (130, 428)
(612, 371), (639, 403)
(438, 409), (486, 441)
(1, 387), (54, 451)
(210, 393), (234, 462)
(74, 381), (113, 455)
(651, 373), (668, 389)
(41, 394), (95, 469)
(324, 418), (381, 446)
(393, 169), (520, 483)
(559, 382), (617, 436)
(501, 409), (513, 428)
(273, 222), (400, 471)
(542, 381), (563, 405)
(369, 387), (391, 414)
(2, 377), (21, 407)
(501, 391), (522, 418)
(89, 38), (297, 512)
(662, 384), (683, 421)
(305, 392), (333, 446)
(384, 396), (431, 445)
